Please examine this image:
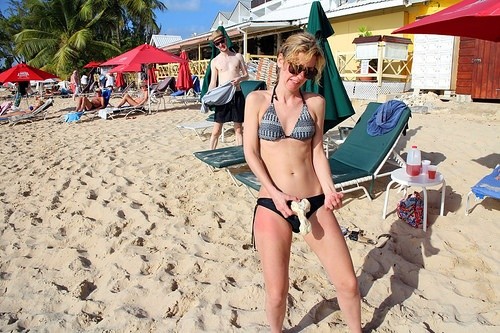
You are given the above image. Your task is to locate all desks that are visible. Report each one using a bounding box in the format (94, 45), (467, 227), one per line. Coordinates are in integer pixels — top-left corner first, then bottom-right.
(382, 166), (446, 232)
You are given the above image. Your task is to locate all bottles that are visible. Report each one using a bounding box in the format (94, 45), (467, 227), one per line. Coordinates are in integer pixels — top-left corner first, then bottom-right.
(406, 145), (421, 177)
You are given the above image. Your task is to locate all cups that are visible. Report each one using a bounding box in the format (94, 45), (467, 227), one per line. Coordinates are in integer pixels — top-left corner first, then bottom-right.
(422, 159), (432, 174)
(427, 165), (438, 181)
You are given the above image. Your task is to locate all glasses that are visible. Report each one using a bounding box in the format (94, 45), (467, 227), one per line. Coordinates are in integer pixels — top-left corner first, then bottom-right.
(284, 56), (318, 79)
(214, 38), (225, 46)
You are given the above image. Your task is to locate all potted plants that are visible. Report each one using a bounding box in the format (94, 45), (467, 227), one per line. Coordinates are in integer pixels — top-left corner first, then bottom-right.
(357, 26), (378, 80)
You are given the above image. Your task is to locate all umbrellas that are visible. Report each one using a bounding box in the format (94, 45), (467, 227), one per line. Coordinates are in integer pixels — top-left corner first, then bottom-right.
(100, 42), (191, 115)
(176, 49), (195, 104)
(115, 72), (126, 93)
(200, 21), (236, 145)
(298, 2), (357, 138)
(392, 1), (500, 42)
(1, 61), (62, 108)
(85, 61), (105, 73)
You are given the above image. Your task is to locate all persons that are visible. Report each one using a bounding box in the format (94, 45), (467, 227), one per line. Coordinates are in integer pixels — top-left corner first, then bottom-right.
(114, 85), (151, 109)
(108, 62), (161, 90)
(241, 32), (363, 333)
(1, 100), (46, 118)
(2, 68), (110, 96)
(206, 29), (250, 151)
(74, 88), (106, 112)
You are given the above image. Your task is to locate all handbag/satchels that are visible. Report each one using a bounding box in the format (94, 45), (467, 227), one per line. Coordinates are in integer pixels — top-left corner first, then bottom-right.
(396, 191), (427, 228)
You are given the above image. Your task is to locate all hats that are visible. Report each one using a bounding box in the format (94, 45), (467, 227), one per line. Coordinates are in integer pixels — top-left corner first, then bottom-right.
(211, 31), (226, 43)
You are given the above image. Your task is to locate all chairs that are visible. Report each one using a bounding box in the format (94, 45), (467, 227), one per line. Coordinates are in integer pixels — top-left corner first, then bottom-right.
(99, 90), (154, 120)
(180, 81), (267, 143)
(0, 102), (11, 115)
(235, 102), (411, 201)
(67, 88), (112, 122)
(8, 98), (53, 128)
(171, 76), (200, 108)
(466, 164), (500, 215)
(194, 145), (246, 187)
